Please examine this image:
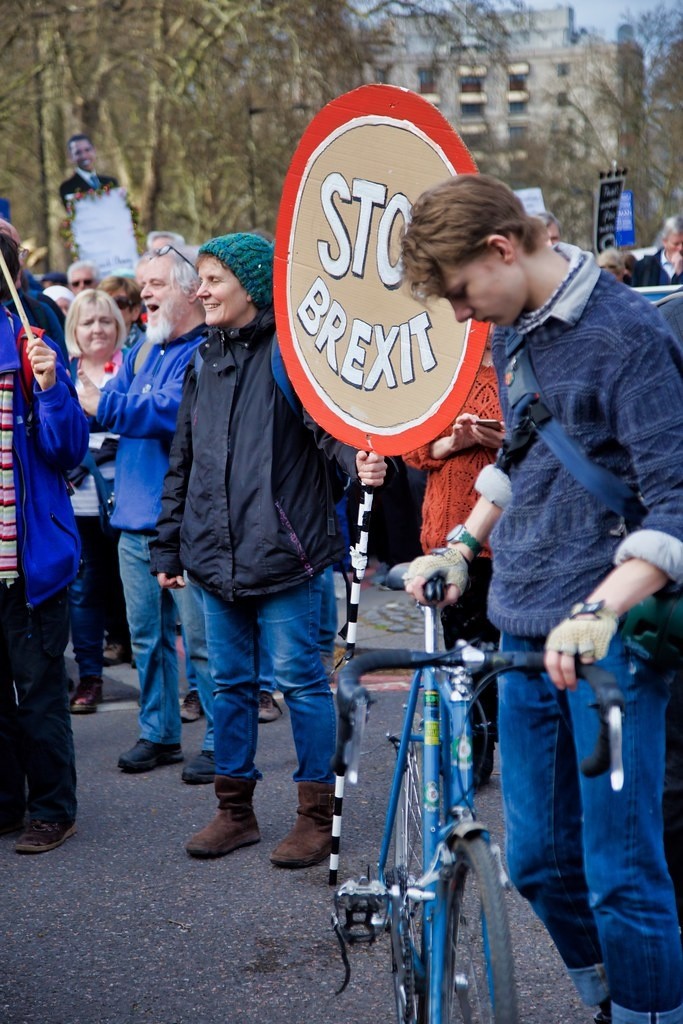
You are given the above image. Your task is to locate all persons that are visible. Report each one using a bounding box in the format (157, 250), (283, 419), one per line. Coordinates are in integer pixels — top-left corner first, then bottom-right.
(78, 246), (221, 784)
(400, 174), (683, 1024)
(148, 233), (388, 867)
(59, 134), (138, 216)
(396, 329), (506, 794)
(0, 233), (91, 853)
(65, 289), (131, 715)
(0, 197), (427, 722)
(528, 205), (683, 347)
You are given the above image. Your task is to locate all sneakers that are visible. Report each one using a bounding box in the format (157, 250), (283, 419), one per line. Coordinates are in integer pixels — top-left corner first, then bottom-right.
(117, 737), (183, 771)
(256, 691), (282, 721)
(182, 747), (220, 785)
(179, 690), (204, 721)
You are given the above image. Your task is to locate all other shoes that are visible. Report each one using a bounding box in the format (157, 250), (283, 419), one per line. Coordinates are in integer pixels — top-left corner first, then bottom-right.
(101, 643), (128, 666)
(473, 747), (497, 791)
(14, 817), (78, 852)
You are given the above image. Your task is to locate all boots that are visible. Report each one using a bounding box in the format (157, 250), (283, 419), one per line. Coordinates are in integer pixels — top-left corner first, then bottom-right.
(269, 782), (336, 867)
(185, 774), (261, 859)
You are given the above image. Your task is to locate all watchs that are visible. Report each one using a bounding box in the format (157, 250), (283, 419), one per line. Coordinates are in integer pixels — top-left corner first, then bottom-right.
(446, 524), (484, 554)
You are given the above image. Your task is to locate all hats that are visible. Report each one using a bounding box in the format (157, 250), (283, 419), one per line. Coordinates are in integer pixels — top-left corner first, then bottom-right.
(42, 285), (75, 305)
(199, 232), (278, 309)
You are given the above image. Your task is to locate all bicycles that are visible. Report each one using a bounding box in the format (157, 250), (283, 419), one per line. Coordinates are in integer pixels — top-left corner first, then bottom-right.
(331, 563), (625, 1024)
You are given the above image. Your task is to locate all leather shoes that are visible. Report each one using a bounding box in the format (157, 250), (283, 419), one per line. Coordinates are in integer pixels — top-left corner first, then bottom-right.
(70, 674), (104, 712)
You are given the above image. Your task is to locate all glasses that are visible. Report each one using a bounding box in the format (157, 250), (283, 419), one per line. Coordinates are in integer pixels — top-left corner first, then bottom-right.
(149, 245), (195, 269)
(71, 276), (98, 288)
(114, 295), (133, 311)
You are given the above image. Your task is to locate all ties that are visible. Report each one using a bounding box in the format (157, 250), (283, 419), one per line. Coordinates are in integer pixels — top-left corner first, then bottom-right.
(90, 175), (101, 189)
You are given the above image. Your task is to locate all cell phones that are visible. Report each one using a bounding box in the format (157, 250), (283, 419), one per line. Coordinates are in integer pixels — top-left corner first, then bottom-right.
(475, 420), (502, 432)
